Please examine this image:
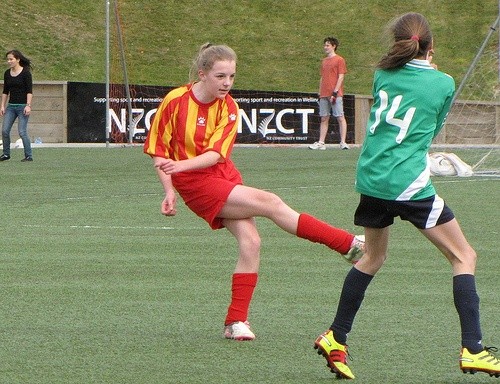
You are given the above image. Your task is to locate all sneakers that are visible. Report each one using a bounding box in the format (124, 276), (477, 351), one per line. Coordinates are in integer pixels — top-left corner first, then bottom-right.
(343, 234), (365, 264)
(313, 329), (355, 379)
(460, 346), (500, 379)
(223, 320), (256, 340)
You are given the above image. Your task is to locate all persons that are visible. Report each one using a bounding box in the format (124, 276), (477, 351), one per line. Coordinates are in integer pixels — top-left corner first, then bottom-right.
(143, 41), (366, 340)
(307, 36), (351, 150)
(314, 11), (500, 380)
(0, 49), (33, 162)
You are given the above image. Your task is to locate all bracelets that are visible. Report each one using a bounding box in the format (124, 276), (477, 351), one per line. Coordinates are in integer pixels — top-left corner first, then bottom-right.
(26, 104), (32, 107)
(332, 92), (337, 98)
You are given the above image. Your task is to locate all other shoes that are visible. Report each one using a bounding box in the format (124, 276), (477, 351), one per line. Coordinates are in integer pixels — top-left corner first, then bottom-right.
(308, 142), (326, 150)
(0, 154), (10, 161)
(21, 156), (33, 162)
(340, 142), (349, 149)
